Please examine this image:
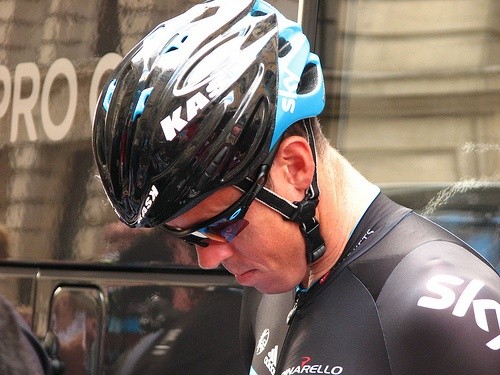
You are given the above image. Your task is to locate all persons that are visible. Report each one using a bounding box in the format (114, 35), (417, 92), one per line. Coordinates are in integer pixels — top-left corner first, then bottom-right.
(1, 224), (52, 375)
(90, 0), (500, 374)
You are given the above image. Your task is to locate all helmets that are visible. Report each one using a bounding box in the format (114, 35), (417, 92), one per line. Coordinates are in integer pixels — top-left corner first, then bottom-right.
(90, 0), (328, 231)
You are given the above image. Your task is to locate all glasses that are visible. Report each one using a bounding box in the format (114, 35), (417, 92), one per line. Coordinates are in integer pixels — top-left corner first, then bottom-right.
(162, 165), (269, 249)
